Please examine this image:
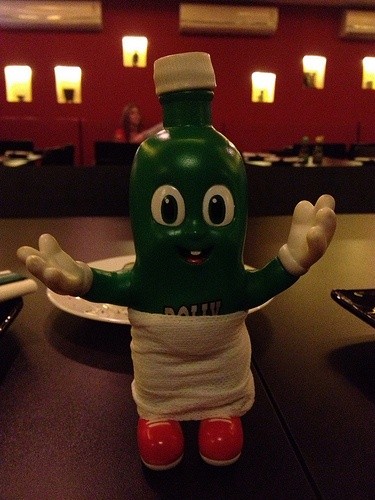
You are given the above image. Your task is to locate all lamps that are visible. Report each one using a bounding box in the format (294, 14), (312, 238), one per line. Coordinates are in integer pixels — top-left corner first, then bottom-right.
(122, 36), (148, 68)
(54, 66), (82, 104)
(362, 57), (375, 91)
(251, 71), (275, 103)
(301, 55), (327, 90)
(4, 65), (32, 103)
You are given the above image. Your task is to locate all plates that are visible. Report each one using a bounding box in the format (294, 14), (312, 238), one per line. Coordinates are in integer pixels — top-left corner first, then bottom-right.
(46, 254), (276, 325)
(0, 296), (25, 332)
(330, 287), (375, 327)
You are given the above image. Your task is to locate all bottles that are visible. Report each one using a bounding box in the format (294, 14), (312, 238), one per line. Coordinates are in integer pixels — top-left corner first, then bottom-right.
(314, 137), (324, 163)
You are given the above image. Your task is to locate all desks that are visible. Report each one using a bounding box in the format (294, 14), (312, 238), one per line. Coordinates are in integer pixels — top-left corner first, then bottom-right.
(0, 151), (41, 218)
(0, 215), (375, 500)
(242, 151), (364, 216)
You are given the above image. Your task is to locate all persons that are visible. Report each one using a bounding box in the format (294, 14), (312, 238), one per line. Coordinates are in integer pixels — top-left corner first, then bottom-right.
(110, 103), (163, 144)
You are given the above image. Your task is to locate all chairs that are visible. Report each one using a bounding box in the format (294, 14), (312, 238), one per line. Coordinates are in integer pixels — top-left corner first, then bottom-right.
(92, 137), (140, 216)
(36, 144), (76, 216)
